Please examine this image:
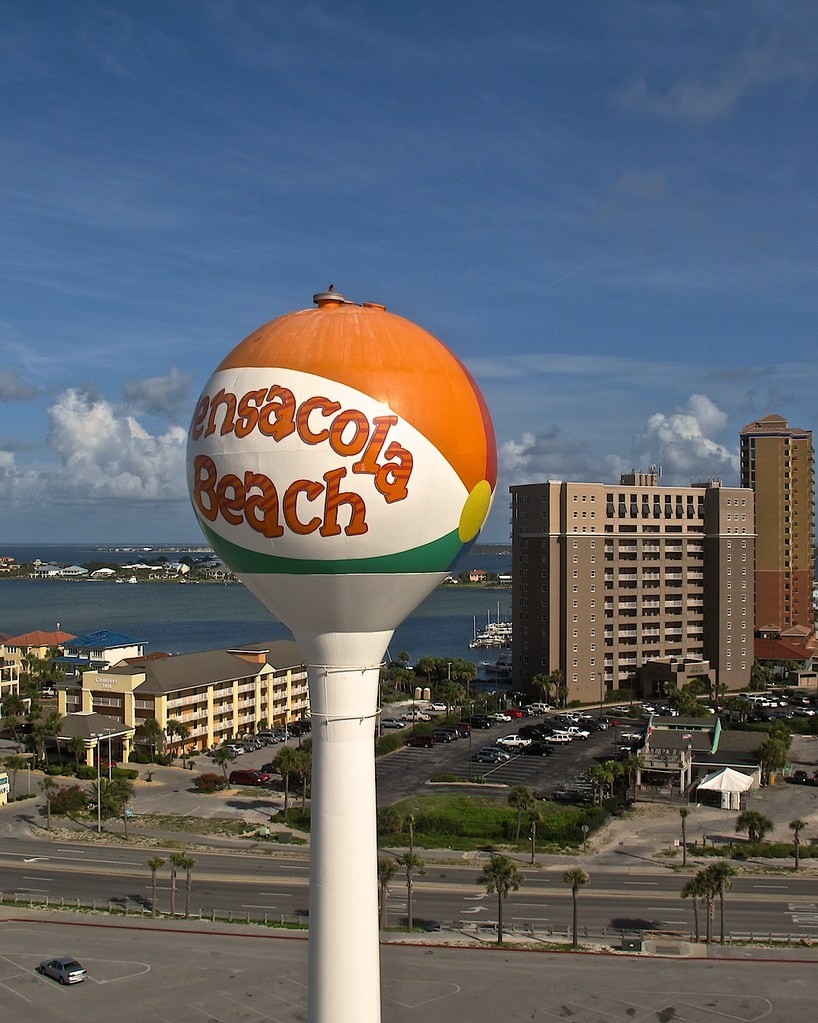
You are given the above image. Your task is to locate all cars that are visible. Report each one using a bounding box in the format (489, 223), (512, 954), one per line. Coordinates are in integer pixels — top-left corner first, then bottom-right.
(793, 770), (807, 785)
(81, 756), (116, 770)
(229, 769), (270, 786)
(639, 703), (680, 717)
(812, 770), (818, 786)
(40, 956), (89, 985)
(433, 703), (612, 764)
(739, 692), (816, 723)
(612, 706), (630, 713)
(226, 718), (314, 757)
(400, 711), (430, 723)
(376, 717), (406, 728)
(703, 706), (715, 715)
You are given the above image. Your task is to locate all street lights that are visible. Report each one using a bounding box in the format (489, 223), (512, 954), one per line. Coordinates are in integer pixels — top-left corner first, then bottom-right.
(90, 732), (104, 834)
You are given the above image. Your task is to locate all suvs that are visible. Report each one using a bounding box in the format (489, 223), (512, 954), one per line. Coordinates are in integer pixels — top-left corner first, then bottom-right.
(429, 703), (453, 712)
(404, 735), (437, 748)
